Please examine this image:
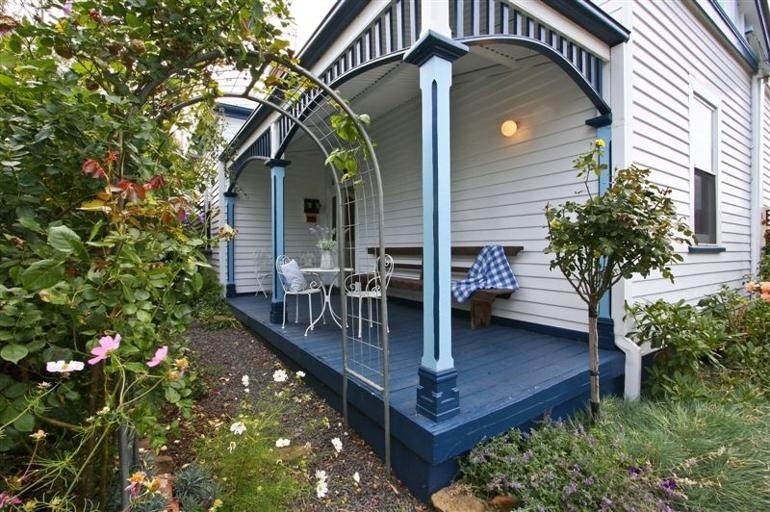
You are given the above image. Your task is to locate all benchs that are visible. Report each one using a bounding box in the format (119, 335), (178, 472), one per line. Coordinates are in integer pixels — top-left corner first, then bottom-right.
(356, 245), (525, 329)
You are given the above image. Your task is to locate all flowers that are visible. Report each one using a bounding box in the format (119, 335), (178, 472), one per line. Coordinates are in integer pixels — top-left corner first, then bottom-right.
(316, 238), (336, 251)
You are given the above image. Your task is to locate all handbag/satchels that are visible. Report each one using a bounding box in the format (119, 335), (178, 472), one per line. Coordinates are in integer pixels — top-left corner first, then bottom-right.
(279, 256), (308, 293)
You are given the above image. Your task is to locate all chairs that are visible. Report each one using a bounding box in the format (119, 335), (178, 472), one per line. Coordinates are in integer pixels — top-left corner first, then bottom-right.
(343, 254), (395, 339)
(254, 250), (326, 331)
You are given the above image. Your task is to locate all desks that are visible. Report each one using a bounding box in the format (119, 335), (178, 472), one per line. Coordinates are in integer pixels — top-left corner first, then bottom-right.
(300, 265), (354, 336)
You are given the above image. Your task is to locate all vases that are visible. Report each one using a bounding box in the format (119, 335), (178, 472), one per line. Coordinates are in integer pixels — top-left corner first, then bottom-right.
(320, 250), (335, 270)
